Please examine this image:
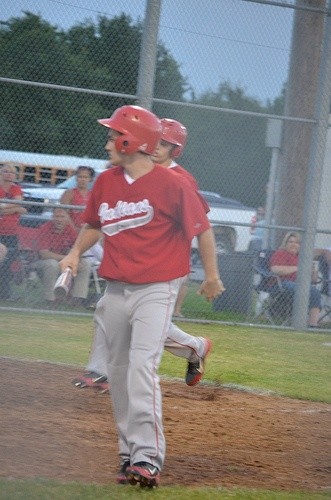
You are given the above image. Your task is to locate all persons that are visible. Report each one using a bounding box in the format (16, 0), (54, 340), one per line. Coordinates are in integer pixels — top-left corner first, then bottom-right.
(173, 274), (188, 318)
(60, 166), (104, 305)
(56, 104), (226, 490)
(0, 162), (27, 297)
(71, 118), (211, 393)
(34, 207), (91, 308)
(269, 231), (323, 327)
(251, 205), (265, 234)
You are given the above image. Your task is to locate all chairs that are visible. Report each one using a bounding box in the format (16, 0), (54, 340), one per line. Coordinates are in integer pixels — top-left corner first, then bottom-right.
(253, 250), (291, 326)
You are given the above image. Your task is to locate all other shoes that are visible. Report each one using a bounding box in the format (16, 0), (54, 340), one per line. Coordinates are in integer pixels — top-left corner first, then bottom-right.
(48, 301), (59, 310)
(65, 301), (76, 309)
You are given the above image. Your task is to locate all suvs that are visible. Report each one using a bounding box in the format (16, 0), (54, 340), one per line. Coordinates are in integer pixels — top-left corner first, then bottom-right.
(17, 169), (259, 255)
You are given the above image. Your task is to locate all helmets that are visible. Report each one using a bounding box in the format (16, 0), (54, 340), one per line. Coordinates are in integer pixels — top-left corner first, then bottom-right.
(160, 118), (187, 158)
(97, 106), (163, 155)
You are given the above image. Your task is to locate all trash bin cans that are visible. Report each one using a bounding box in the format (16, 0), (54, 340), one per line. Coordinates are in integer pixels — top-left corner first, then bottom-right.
(213, 251), (256, 314)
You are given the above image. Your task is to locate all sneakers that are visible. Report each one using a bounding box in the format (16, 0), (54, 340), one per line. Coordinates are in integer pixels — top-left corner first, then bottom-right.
(72, 372), (110, 394)
(186, 337), (211, 386)
(115, 462), (131, 484)
(126, 462), (160, 490)
